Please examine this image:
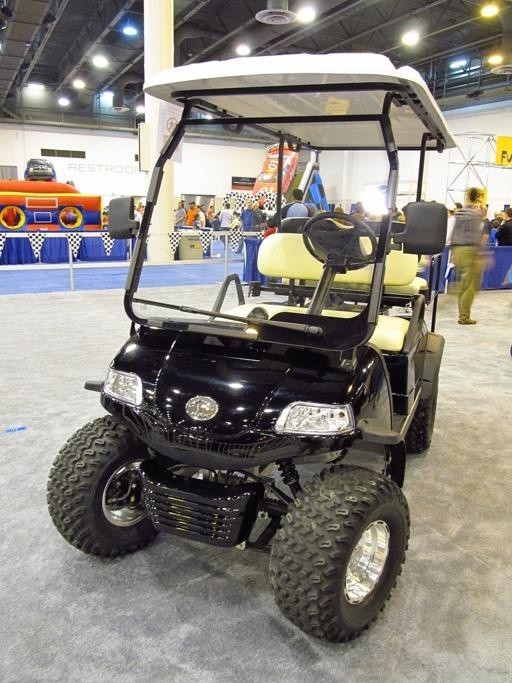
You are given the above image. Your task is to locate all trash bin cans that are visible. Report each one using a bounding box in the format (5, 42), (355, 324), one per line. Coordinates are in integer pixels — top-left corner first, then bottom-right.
(178, 235), (203, 260)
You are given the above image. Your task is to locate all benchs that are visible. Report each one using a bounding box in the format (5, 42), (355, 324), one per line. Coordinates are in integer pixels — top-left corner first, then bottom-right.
(220, 232), (425, 356)
(279, 217), (431, 304)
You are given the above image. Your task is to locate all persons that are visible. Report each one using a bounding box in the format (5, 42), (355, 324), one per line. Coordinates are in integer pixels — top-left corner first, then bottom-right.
(171, 196), (268, 232)
(281, 188), (311, 216)
(448, 197), (484, 324)
(444, 202), (511, 249)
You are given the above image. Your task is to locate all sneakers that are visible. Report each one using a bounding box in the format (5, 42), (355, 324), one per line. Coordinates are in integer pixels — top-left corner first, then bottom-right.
(458, 317), (477, 324)
